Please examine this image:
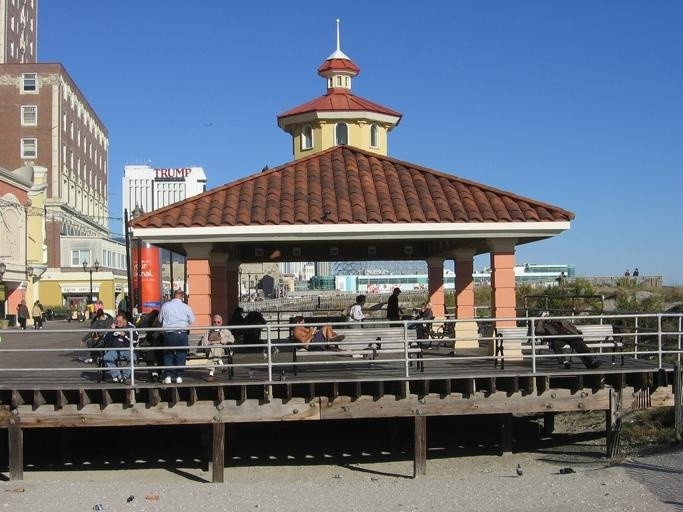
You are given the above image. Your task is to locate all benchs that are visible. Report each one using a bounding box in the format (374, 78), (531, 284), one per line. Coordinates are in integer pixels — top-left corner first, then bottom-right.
(285, 327), (426, 371)
(92, 334), (236, 383)
(492, 318), (626, 369)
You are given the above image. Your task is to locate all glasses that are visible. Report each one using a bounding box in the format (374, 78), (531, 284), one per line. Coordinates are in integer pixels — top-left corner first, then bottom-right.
(213, 321), (221, 324)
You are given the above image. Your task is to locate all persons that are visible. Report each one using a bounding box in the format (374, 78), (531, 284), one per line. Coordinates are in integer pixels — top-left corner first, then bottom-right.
(80, 289), (235, 384)
(535, 308), (602, 369)
(346, 295), (366, 329)
(294, 316), (345, 351)
(16, 299), (43, 330)
(386, 288), (433, 339)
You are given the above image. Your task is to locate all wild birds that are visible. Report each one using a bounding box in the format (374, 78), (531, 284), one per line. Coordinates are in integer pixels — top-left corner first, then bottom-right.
(279, 369), (288, 382)
(248, 367), (255, 380)
(320, 210), (332, 223)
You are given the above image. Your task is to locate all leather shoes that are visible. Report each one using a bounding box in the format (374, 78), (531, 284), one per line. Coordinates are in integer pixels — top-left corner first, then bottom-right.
(207, 375), (214, 383)
(563, 362), (571, 369)
(222, 367), (228, 375)
(587, 360), (601, 369)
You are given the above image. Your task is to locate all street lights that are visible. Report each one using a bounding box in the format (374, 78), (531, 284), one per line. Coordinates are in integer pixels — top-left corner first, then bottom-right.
(123, 200), (145, 326)
(238, 266), (242, 302)
(82, 259), (100, 304)
(254, 272), (258, 301)
(247, 271), (251, 301)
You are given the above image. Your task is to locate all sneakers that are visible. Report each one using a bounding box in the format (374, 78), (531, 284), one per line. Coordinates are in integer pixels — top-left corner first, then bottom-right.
(162, 376), (171, 384)
(112, 376), (128, 383)
(175, 377), (182, 384)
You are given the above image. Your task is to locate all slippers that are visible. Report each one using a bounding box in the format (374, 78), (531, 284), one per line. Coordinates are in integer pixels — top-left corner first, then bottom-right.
(328, 335), (345, 341)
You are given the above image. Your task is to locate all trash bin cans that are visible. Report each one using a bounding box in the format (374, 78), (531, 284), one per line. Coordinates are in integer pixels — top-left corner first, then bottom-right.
(6, 314), (16, 327)
(47, 309), (55, 321)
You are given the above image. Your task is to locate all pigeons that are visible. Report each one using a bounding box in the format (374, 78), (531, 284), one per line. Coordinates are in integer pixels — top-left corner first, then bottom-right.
(262, 163), (269, 173)
(516, 463), (523, 477)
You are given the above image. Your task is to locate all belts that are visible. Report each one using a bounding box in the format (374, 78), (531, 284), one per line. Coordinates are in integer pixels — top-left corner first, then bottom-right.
(163, 331), (187, 334)
